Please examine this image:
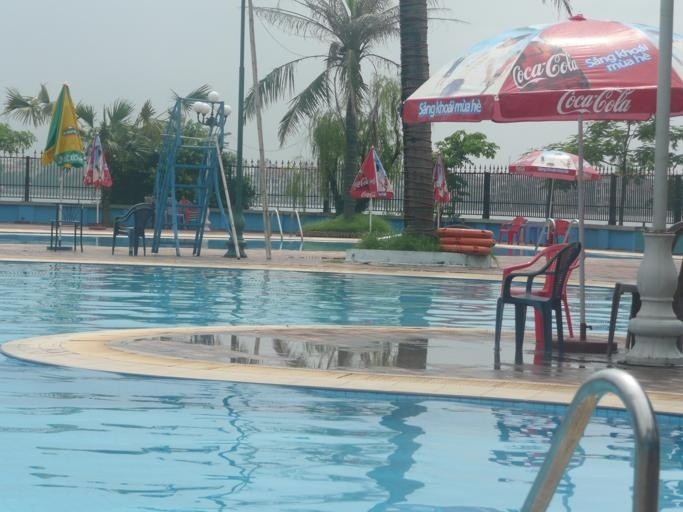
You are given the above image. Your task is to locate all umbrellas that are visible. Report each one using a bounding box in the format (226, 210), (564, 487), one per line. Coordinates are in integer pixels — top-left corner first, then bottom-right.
(40, 80), (86, 228)
(82, 132), (114, 224)
(400, 12), (683, 339)
(432, 151), (451, 229)
(348, 145), (395, 232)
(508, 149), (603, 240)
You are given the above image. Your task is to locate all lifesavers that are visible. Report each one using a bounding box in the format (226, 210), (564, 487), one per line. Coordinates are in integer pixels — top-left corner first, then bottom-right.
(437, 229), (497, 255)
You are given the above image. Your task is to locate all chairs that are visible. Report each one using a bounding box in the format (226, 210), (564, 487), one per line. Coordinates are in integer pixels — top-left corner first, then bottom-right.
(112, 195), (203, 257)
(499, 215), (570, 247)
(50, 202), (84, 253)
(607, 220), (683, 354)
(501, 243), (585, 352)
(494, 241), (583, 370)
